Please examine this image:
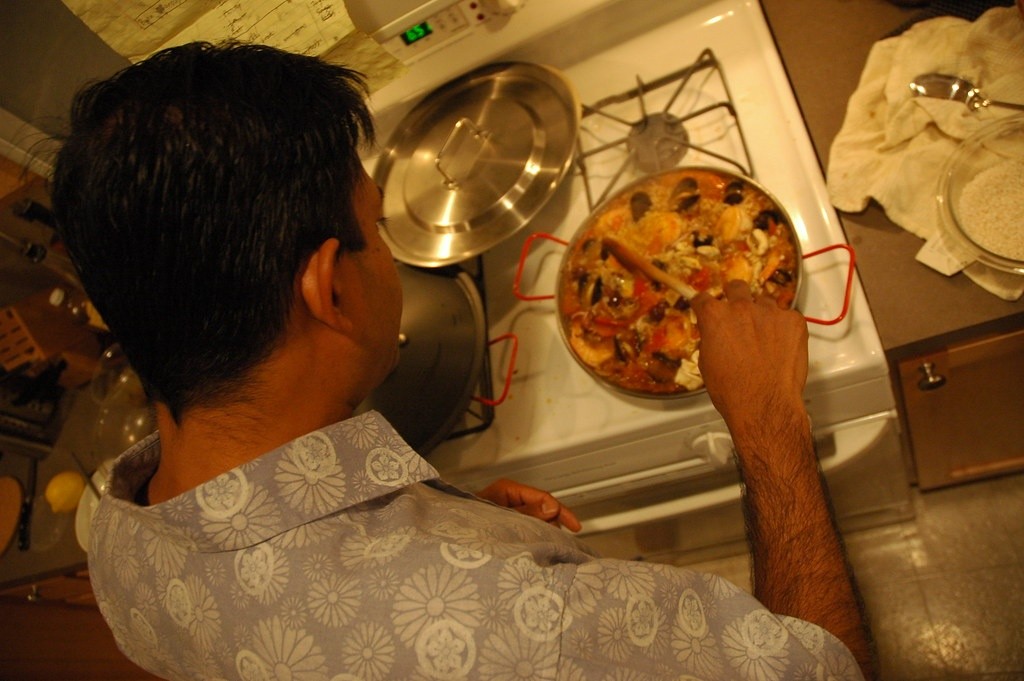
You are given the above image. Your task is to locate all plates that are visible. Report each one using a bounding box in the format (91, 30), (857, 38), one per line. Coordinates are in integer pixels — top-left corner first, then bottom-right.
(74, 459), (113, 552)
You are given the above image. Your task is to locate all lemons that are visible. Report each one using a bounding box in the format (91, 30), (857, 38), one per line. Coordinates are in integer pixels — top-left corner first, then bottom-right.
(45, 470), (85, 513)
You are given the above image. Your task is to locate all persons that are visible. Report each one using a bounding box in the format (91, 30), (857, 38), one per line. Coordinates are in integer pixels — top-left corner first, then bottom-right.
(52, 40), (878, 681)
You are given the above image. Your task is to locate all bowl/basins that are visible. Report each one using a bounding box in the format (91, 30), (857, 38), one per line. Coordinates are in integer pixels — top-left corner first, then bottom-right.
(935, 116), (1024, 289)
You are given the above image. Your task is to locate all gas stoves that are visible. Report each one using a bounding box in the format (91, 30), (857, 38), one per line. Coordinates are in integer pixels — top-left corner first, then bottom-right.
(348, 3), (896, 505)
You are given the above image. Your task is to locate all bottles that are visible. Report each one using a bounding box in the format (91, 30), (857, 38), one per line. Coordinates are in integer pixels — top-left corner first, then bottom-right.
(23, 242), (87, 294)
(49, 289), (111, 335)
(14, 197), (56, 229)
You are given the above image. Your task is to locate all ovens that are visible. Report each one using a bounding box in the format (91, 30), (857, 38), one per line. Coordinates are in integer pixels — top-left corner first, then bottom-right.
(547, 408), (916, 566)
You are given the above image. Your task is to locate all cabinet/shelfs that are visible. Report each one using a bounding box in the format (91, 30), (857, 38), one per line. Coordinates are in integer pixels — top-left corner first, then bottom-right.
(890, 325), (1024, 493)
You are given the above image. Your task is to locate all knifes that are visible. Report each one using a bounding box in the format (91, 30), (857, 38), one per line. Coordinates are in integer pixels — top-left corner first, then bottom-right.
(17, 458), (36, 551)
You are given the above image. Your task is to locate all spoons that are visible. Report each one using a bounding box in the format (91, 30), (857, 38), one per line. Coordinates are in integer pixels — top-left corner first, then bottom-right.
(909, 72), (1024, 114)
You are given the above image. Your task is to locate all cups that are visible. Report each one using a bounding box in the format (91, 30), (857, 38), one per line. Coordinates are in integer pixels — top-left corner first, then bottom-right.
(86, 344), (155, 476)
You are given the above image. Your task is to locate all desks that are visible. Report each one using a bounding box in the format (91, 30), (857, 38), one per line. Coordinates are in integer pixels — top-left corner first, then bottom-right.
(0, 177), (156, 613)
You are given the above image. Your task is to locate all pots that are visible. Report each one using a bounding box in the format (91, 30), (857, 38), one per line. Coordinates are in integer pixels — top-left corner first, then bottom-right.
(389, 259), (519, 457)
(512, 168), (855, 401)
